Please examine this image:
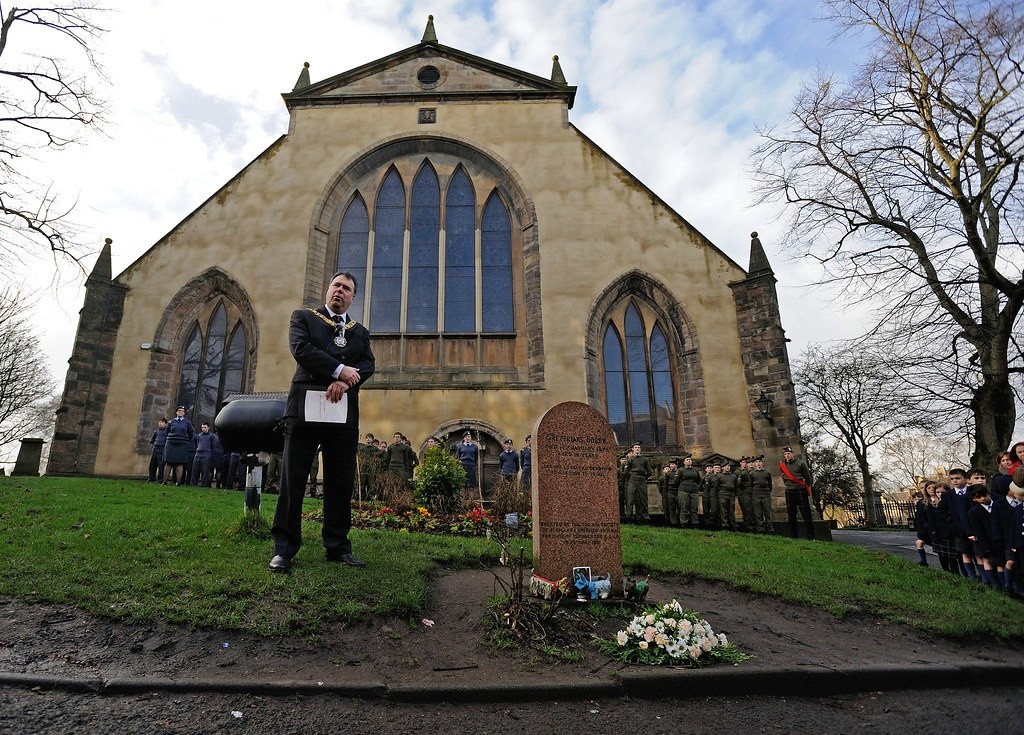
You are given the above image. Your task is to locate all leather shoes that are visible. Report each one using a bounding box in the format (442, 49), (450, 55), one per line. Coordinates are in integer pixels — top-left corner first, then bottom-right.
(325, 552), (367, 566)
(268, 554), (294, 574)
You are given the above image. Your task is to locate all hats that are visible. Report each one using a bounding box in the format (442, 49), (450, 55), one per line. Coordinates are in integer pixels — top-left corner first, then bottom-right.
(1009, 465), (1024, 494)
(619, 442), (729, 469)
(175, 406), (185, 412)
(782, 445), (792, 453)
(739, 457), (746, 462)
(746, 458), (754, 463)
(754, 457), (762, 462)
(1009, 441), (1024, 464)
(526, 435), (531, 443)
(505, 439), (512, 444)
(461, 431), (470, 438)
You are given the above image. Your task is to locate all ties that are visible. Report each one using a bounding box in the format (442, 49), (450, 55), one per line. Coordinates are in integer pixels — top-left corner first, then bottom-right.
(178, 416), (181, 422)
(508, 450), (510, 454)
(959, 491), (964, 495)
(333, 315), (343, 325)
(988, 506), (991, 513)
(529, 449), (531, 452)
(1011, 501), (1016, 507)
(467, 442), (469, 447)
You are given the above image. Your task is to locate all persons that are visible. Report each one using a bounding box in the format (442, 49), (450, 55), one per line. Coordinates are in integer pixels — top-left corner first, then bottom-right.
(268, 272), (375, 572)
(702, 461), (739, 528)
(147, 406), (246, 491)
(498, 439), (520, 483)
(424, 431), (478, 500)
(658, 456), (702, 524)
(617, 442), (653, 522)
(351, 432), (420, 502)
(738, 457), (775, 531)
(262, 444), (323, 498)
(912, 441), (1024, 605)
(781, 446), (816, 540)
(519, 435), (531, 488)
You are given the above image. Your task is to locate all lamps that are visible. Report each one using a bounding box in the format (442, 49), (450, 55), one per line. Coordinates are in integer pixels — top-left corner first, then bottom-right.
(214, 399), (287, 464)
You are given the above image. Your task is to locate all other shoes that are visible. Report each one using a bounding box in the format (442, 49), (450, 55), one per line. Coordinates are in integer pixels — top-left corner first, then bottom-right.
(917, 560), (929, 567)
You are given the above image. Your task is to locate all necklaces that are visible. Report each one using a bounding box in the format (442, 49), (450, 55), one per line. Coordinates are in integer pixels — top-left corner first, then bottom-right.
(307, 308), (356, 347)
